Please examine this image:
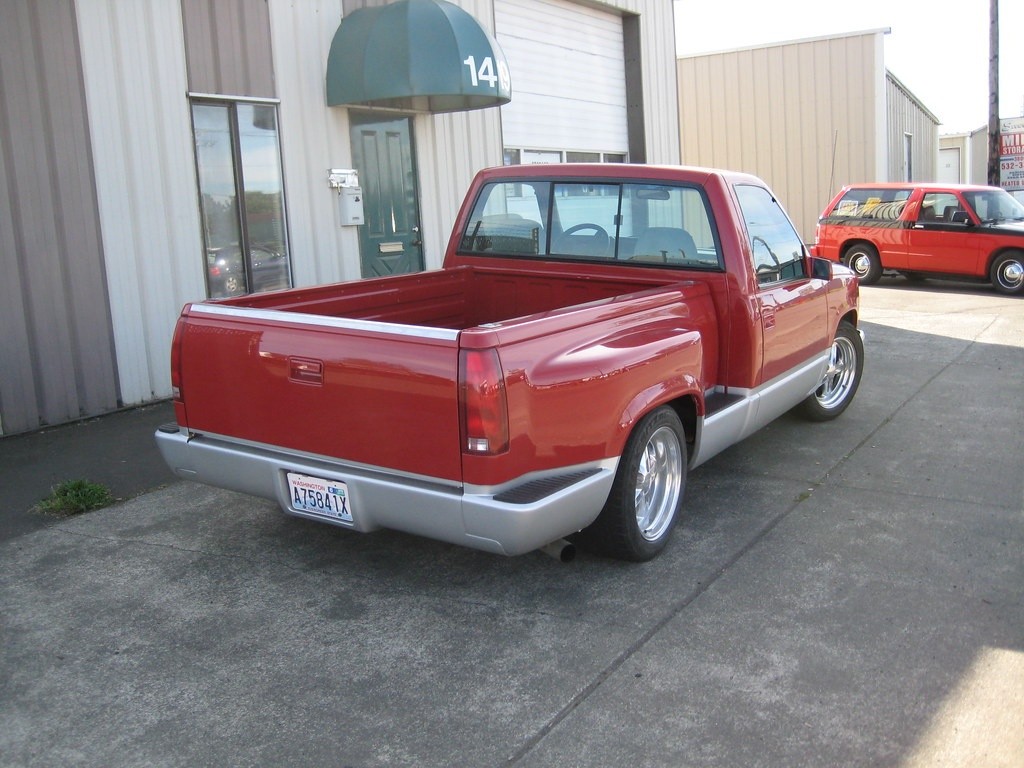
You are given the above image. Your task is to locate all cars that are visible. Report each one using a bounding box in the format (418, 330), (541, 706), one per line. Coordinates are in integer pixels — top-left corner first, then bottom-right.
(206, 243), (289, 297)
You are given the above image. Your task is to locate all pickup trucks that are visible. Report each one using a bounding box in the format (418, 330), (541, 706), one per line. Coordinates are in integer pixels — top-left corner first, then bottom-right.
(152, 161), (867, 565)
(808, 182), (1024, 296)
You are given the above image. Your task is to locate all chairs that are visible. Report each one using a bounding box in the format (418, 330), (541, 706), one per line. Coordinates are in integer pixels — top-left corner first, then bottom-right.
(920, 206), (937, 220)
(493, 220), (546, 254)
(633, 229), (699, 258)
(943, 206), (960, 220)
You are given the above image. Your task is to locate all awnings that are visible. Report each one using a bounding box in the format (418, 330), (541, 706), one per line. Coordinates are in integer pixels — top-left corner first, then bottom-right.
(326, 0), (512, 114)
